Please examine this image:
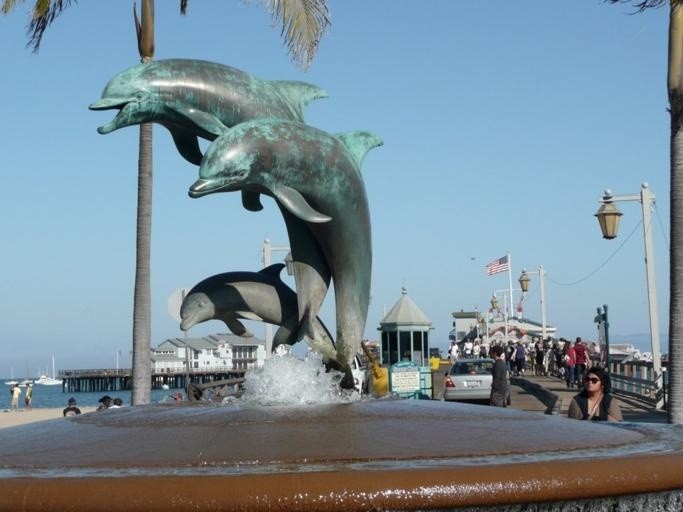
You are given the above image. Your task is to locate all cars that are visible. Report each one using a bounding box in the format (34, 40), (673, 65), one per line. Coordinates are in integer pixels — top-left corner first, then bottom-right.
(350, 354), (368, 393)
(443, 357), (512, 408)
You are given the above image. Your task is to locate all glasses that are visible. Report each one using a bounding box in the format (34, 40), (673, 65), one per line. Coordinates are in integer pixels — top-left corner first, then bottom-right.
(585, 376), (599, 383)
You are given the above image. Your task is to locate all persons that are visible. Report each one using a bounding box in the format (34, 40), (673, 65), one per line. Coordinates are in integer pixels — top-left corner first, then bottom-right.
(567, 365), (623, 421)
(95, 395), (111, 412)
(8, 382), (22, 412)
(172, 393), (183, 402)
(447, 334), (593, 390)
(22, 382), (34, 413)
(63, 397), (81, 418)
(106, 398), (125, 411)
(488, 345), (511, 408)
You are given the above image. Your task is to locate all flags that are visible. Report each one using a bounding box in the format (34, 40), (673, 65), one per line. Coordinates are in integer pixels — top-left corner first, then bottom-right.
(485, 255), (509, 276)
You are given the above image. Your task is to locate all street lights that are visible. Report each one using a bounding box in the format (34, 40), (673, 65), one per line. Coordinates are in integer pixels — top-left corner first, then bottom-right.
(591, 178), (662, 387)
(490, 286), (510, 345)
(517, 264), (547, 340)
(261, 238), (294, 359)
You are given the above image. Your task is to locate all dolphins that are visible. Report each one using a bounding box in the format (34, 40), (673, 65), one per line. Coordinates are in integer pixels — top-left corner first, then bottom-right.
(187, 115), (385, 372)
(86, 58), (331, 344)
(178, 263), (342, 366)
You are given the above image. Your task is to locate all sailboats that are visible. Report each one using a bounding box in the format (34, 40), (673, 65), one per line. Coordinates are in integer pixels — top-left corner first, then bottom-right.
(4, 355), (62, 387)
